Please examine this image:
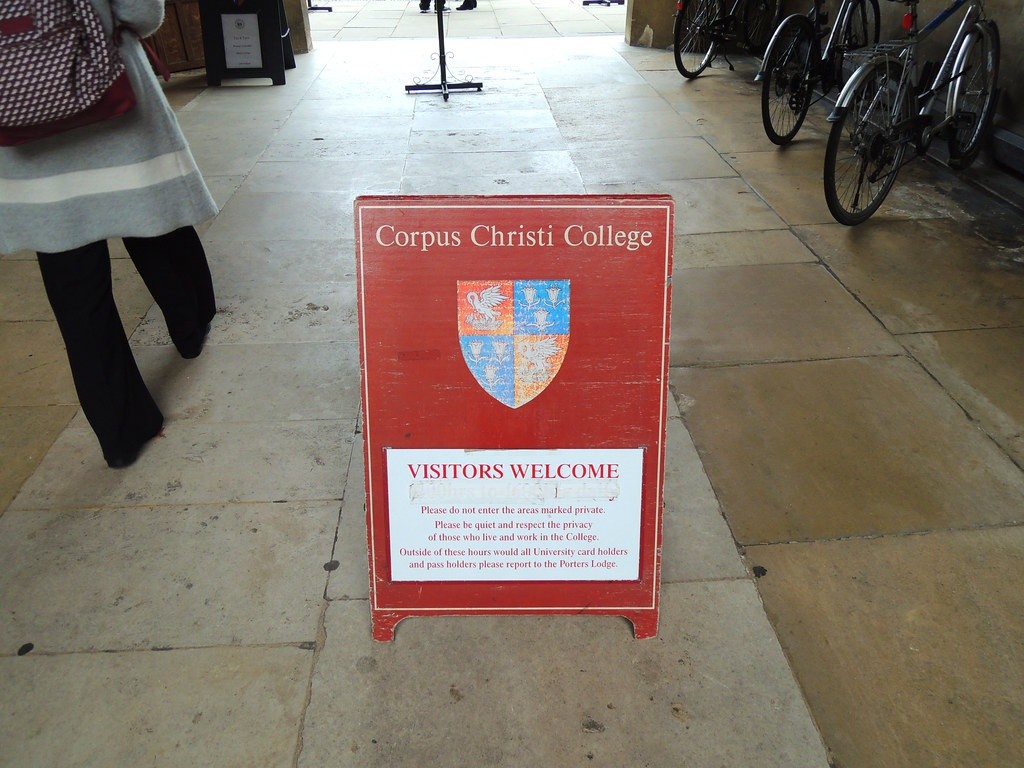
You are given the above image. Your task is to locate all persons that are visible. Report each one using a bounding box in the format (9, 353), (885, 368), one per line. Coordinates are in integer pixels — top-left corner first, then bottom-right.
(0, 0), (217, 471)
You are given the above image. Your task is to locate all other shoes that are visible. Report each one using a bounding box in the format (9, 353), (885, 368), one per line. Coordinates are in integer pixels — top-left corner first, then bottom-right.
(434, 5), (451, 13)
(455, 0), (477, 10)
(180, 323), (212, 359)
(104, 443), (143, 468)
(420, 6), (431, 13)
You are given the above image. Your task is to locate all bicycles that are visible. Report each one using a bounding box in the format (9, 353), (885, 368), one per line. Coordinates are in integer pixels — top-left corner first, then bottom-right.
(756, 0), (884, 146)
(815, 0), (1004, 228)
(672, 0), (780, 82)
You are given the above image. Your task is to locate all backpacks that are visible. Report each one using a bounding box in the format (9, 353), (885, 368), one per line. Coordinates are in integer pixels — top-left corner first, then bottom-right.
(0, 1), (140, 151)
